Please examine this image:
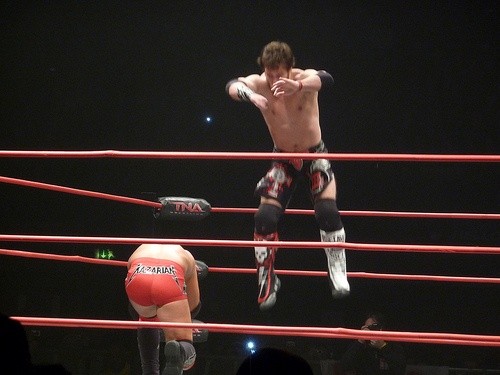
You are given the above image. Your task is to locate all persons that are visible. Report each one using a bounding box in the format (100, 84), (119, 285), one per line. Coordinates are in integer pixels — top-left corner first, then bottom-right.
(225, 41), (352, 309)
(125, 243), (202, 375)
(338, 311), (406, 375)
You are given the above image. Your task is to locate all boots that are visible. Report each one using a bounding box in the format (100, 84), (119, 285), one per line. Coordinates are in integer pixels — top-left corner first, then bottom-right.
(253, 232), (279, 309)
(162, 339), (196, 375)
(319, 227), (351, 297)
(136, 328), (161, 375)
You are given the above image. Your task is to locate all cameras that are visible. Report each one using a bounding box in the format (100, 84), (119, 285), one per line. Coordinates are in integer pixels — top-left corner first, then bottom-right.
(368, 323), (382, 331)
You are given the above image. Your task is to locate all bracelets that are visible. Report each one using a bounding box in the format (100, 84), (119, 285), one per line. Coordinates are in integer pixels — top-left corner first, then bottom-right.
(298, 80), (303, 92)
